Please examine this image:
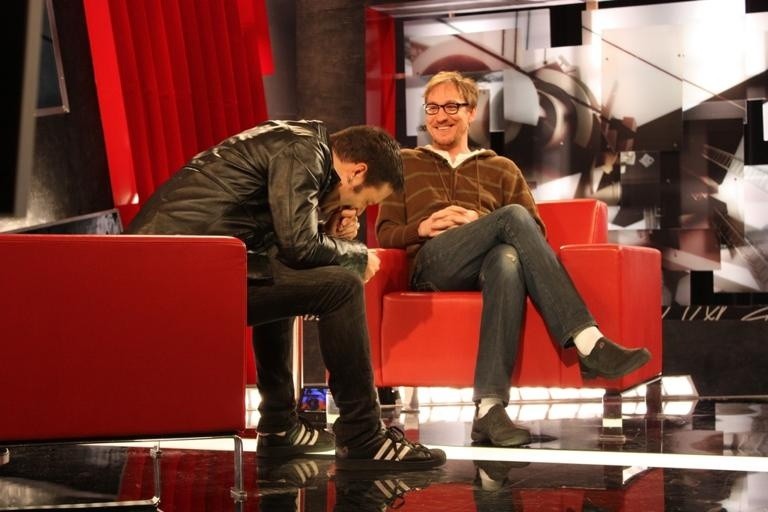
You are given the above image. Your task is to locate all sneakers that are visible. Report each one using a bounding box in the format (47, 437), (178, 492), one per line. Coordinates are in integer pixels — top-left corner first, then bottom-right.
(254, 458), (332, 489)
(335, 470), (449, 503)
(333, 420), (446, 470)
(256, 416), (336, 458)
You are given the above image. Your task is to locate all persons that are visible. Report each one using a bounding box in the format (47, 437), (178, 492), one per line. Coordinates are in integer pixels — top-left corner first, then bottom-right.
(123, 120), (447, 473)
(374, 69), (650, 449)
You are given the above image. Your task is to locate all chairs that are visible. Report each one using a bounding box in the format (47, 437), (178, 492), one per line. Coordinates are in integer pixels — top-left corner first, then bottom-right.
(0, 209), (258, 504)
(363, 199), (663, 404)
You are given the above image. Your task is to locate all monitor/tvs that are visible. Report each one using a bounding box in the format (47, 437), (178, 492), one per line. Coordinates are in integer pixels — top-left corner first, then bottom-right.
(297, 386), (329, 414)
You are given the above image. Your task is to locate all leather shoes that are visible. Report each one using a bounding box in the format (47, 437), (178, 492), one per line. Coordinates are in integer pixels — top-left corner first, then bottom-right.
(575, 337), (649, 377)
(472, 460), (529, 483)
(471, 401), (532, 448)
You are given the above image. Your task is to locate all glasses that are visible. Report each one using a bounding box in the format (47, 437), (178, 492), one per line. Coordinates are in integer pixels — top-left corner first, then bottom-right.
(425, 102), (468, 116)
